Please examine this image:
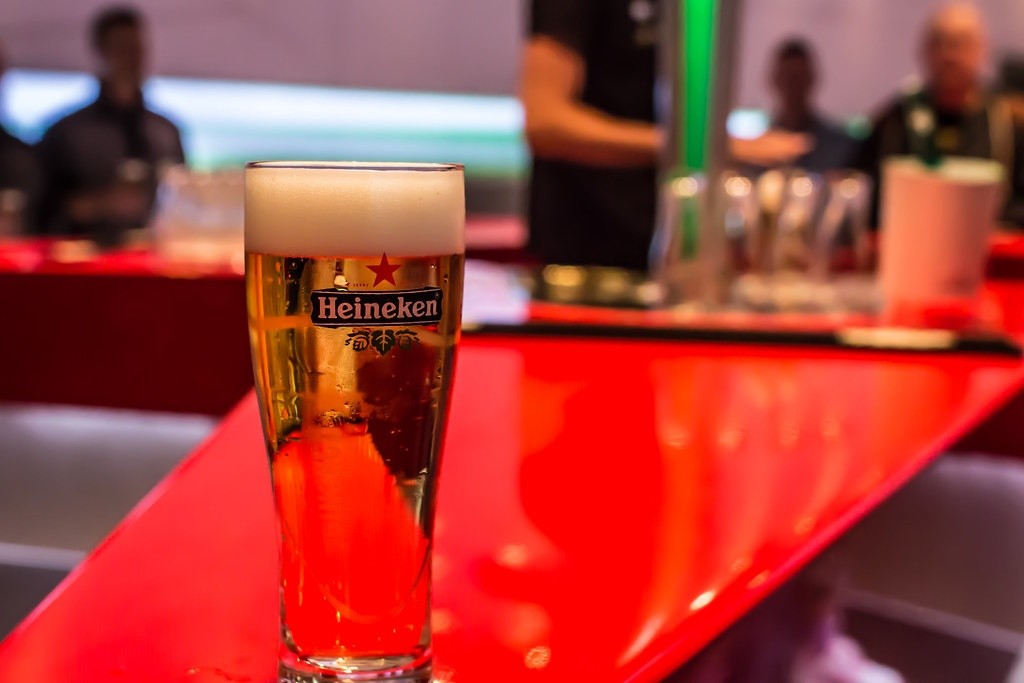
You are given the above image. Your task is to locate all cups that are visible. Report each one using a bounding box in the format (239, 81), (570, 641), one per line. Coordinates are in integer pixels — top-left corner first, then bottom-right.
(242, 159), (468, 683)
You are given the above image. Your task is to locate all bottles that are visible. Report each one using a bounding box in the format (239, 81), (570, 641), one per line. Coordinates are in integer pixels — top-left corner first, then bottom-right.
(905, 107), (951, 171)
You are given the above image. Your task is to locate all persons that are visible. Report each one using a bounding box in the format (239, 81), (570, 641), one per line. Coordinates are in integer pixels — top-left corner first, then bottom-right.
(753, 1), (1024, 248)
(515, 1), (814, 279)
(22, 7), (185, 238)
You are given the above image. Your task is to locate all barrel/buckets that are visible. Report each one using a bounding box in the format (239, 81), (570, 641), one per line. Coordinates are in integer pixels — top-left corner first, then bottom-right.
(877, 155), (1006, 308)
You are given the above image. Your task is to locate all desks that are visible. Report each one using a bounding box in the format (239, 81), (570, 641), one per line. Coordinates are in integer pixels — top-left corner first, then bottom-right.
(0, 338), (1024, 683)
